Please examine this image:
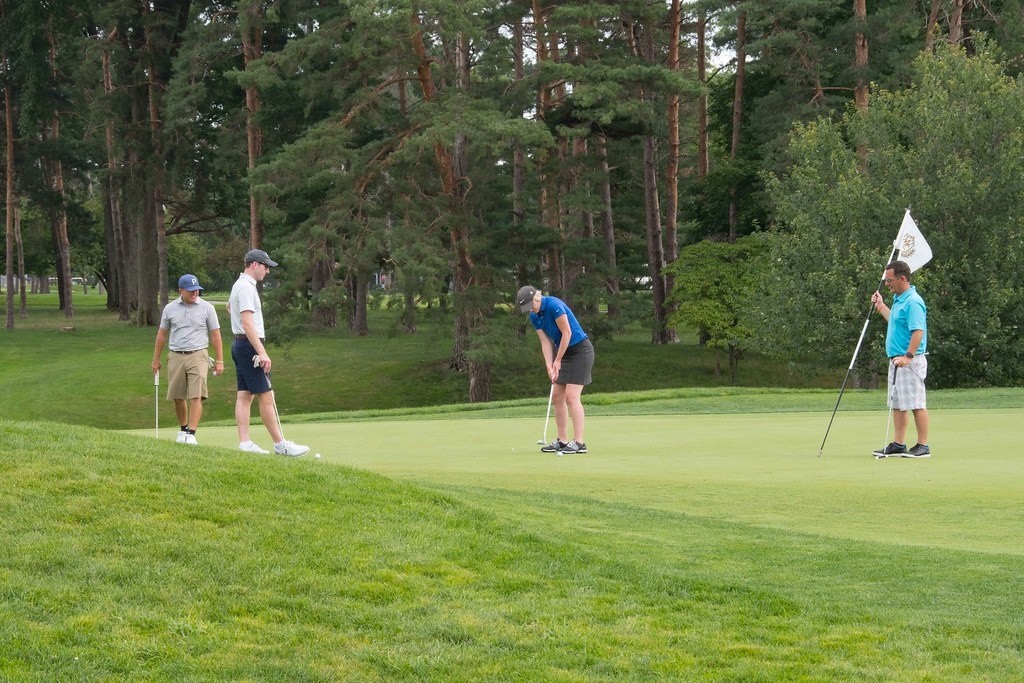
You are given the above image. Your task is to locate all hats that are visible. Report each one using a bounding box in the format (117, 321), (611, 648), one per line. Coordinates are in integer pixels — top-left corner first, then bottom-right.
(178, 274), (205, 292)
(517, 286), (536, 312)
(244, 249), (278, 268)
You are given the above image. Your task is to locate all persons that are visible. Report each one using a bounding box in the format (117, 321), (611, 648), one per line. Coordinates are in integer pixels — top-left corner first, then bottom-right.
(517, 286), (594, 454)
(871, 261), (931, 458)
(225, 250), (310, 458)
(152, 274), (224, 444)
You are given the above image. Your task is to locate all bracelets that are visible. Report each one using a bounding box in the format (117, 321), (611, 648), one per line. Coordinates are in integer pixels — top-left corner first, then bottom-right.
(906, 352), (914, 358)
(216, 361), (223, 364)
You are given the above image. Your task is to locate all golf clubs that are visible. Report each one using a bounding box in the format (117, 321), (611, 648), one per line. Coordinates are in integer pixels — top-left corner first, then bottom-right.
(537, 376), (554, 444)
(878, 366), (897, 459)
(154, 368), (159, 440)
(252, 355), (287, 457)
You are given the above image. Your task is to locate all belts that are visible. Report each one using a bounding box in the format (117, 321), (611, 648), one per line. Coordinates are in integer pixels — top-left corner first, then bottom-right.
(234, 334), (264, 343)
(173, 350), (196, 355)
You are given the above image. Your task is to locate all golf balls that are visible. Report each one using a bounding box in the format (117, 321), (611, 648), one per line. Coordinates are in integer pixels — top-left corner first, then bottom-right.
(894, 359), (899, 364)
(557, 452), (563, 456)
(315, 454), (320, 459)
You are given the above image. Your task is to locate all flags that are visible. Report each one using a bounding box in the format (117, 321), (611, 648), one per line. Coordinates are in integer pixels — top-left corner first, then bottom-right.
(893, 211), (933, 274)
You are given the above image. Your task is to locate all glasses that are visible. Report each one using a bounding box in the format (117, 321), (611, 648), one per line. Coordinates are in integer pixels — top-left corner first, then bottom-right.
(885, 277), (899, 283)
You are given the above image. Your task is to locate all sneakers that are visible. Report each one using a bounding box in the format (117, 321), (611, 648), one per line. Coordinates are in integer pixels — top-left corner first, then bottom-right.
(176, 430), (186, 444)
(561, 439), (588, 454)
(184, 433), (198, 445)
(901, 442), (930, 458)
(238, 443), (269, 456)
(871, 442), (907, 458)
(540, 438), (570, 452)
(273, 440), (310, 458)
